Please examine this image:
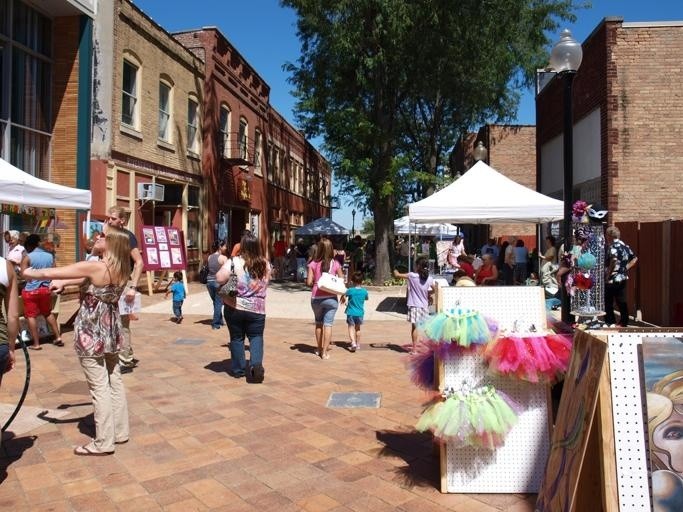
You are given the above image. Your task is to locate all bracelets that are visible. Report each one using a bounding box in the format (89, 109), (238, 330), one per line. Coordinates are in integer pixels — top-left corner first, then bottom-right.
(130, 285), (138, 289)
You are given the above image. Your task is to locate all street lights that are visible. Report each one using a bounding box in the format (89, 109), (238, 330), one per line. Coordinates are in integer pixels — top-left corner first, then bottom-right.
(351, 208), (356, 238)
(470, 142), (487, 251)
(551, 29), (582, 325)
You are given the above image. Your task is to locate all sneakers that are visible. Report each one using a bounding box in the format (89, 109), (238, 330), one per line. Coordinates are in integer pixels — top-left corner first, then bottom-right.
(250, 366), (264, 383)
(121, 366), (133, 374)
(229, 369), (246, 377)
(346, 342), (360, 351)
(314, 349), (330, 359)
(175, 316), (183, 322)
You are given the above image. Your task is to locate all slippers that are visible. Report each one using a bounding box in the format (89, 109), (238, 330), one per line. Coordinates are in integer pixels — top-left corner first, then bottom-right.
(53, 339), (64, 346)
(115, 439), (129, 443)
(75, 442), (114, 456)
(29, 345), (42, 350)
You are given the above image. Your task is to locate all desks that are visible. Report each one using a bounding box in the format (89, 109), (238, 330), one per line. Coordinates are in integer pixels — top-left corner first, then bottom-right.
(1, 292), (61, 344)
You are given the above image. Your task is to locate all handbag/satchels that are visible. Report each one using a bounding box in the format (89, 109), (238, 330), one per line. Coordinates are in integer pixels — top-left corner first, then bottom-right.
(318, 272), (346, 296)
(217, 275), (237, 298)
(200, 264), (208, 284)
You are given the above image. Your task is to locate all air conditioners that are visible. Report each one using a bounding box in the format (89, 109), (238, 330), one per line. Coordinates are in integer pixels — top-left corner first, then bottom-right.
(147, 186), (164, 201)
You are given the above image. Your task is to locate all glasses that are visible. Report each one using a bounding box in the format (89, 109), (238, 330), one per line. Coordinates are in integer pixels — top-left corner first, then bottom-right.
(99, 232), (108, 239)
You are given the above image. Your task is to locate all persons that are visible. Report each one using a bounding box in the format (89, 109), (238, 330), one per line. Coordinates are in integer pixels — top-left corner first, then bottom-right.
(307, 238), (345, 360)
(4, 229), (27, 280)
(101, 207), (144, 373)
(165, 272), (186, 323)
(216, 236), (271, 384)
(205, 239), (231, 331)
(229, 230), (252, 256)
(0, 256), (21, 445)
(24, 228), (131, 457)
(341, 272), (367, 352)
(394, 257), (437, 357)
(604, 227), (639, 328)
(646, 370), (682, 511)
(449, 232), (559, 287)
(20, 234), (64, 350)
(273, 233), (375, 285)
